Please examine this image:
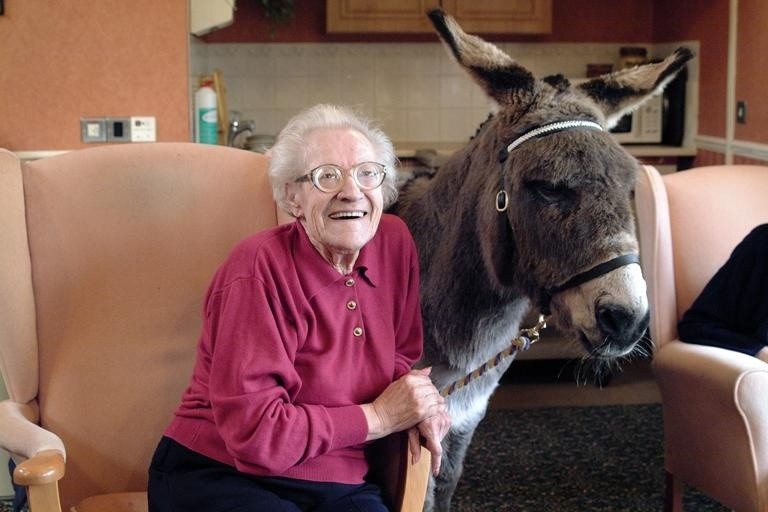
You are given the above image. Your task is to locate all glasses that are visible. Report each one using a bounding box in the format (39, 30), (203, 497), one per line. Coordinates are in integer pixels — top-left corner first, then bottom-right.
(296, 163), (388, 192)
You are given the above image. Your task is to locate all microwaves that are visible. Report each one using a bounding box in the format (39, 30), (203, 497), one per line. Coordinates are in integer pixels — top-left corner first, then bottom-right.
(564, 79), (665, 146)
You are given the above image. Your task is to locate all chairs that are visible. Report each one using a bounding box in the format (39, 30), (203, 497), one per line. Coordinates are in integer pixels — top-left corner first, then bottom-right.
(0, 143), (433, 511)
(633, 165), (768, 512)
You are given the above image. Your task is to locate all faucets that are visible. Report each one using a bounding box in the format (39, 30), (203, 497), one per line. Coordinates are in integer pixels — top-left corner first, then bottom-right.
(226, 109), (257, 149)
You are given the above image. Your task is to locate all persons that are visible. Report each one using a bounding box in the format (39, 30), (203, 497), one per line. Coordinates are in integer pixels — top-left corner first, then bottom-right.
(678, 223), (768, 364)
(147, 102), (451, 511)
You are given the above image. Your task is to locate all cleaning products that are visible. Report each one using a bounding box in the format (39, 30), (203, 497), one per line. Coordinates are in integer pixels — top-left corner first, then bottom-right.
(195, 76), (219, 143)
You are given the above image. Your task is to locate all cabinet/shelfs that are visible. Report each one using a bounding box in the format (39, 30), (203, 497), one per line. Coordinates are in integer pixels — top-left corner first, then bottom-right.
(325, 0), (552, 35)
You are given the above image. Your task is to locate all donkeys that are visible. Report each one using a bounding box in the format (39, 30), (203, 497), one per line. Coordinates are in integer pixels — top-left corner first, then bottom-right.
(382, 5), (697, 512)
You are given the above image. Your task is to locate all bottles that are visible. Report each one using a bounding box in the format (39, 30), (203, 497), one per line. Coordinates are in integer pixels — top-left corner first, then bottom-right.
(194, 80), (220, 145)
(619, 47), (648, 71)
